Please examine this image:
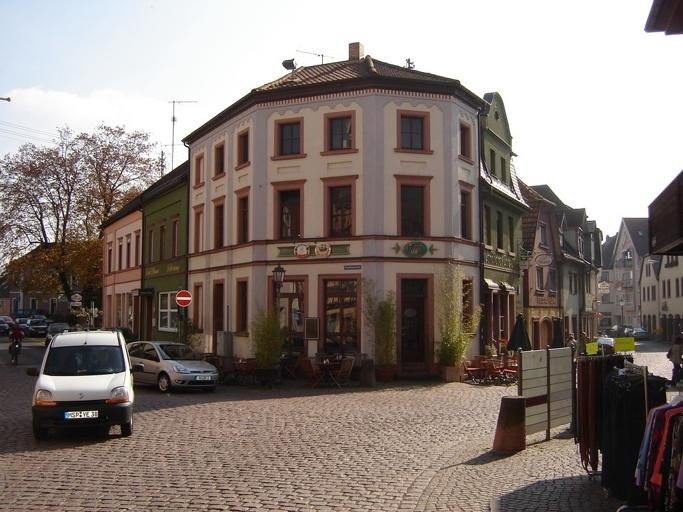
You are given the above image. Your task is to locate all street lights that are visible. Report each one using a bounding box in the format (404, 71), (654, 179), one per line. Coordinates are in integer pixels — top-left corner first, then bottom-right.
(272, 263), (286, 358)
(619, 298), (624, 326)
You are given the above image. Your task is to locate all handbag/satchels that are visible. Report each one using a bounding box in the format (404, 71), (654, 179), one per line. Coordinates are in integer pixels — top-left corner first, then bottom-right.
(667, 349), (671, 357)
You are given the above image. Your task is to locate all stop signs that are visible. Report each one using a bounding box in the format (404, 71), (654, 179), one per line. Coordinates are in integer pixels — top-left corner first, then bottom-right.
(175, 290), (192, 308)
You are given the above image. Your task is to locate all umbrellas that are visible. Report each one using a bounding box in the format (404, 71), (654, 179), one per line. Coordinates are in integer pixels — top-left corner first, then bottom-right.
(505, 313), (533, 353)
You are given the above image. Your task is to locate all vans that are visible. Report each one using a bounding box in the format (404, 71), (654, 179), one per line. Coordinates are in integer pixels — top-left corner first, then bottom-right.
(25, 329), (135, 436)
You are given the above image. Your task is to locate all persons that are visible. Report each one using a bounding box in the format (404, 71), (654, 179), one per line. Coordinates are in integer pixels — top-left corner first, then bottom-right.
(564, 329), (592, 368)
(7, 324), (28, 365)
(667, 336), (683, 388)
(90, 348), (116, 374)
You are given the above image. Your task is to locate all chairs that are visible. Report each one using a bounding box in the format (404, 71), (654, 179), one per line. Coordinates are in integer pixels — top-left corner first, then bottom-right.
(93, 351), (112, 369)
(201, 353), (354, 389)
(55, 348), (76, 372)
(463, 358), (518, 384)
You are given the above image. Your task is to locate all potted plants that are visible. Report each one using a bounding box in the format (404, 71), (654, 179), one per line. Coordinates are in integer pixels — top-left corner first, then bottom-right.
(375, 296), (396, 382)
(437, 273), (480, 382)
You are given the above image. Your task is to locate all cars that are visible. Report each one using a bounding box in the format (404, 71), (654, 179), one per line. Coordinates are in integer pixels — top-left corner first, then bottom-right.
(612, 323), (649, 340)
(127, 339), (220, 393)
(45, 323), (71, 347)
(0, 315), (49, 338)
